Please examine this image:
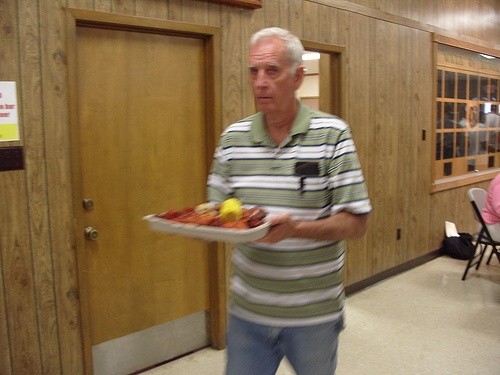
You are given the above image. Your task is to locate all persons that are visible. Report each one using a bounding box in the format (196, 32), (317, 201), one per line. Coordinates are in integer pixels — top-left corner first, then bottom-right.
(479, 173), (500, 242)
(435, 97), (500, 160)
(195, 26), (373, 375)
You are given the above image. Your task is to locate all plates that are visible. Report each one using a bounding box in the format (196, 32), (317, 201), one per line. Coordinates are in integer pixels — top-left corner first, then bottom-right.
(144, 212), (271, 241)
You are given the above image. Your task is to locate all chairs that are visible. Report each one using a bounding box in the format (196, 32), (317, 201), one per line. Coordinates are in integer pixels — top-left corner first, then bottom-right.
(461, 188), (500, 280)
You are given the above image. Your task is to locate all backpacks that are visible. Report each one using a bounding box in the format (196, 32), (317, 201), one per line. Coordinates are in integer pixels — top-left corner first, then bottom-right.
(441, 233), (476, 259)
